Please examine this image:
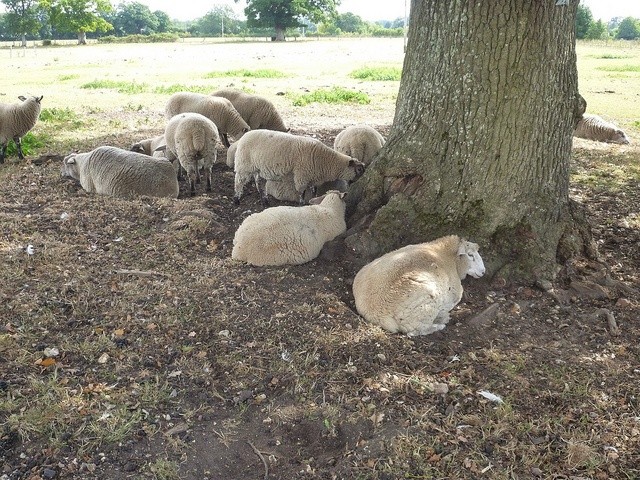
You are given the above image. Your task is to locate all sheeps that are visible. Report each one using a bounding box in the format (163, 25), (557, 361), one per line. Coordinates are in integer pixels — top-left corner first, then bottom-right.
(63, 145), (180, 198)
(155, 112), (220, 195)
(334, 124), (386, 164)
(231, 188), (349, 266)
(266, 178), (349, 203)
(352, 234), (486, 338)
(0, 95), (44, 163)
(210, 88), (290, 135)
(573, 111), (629, 145)
(165, 92), (251, 147)
(227, 128), (365, 210)
(131, 134), (205, 176)
